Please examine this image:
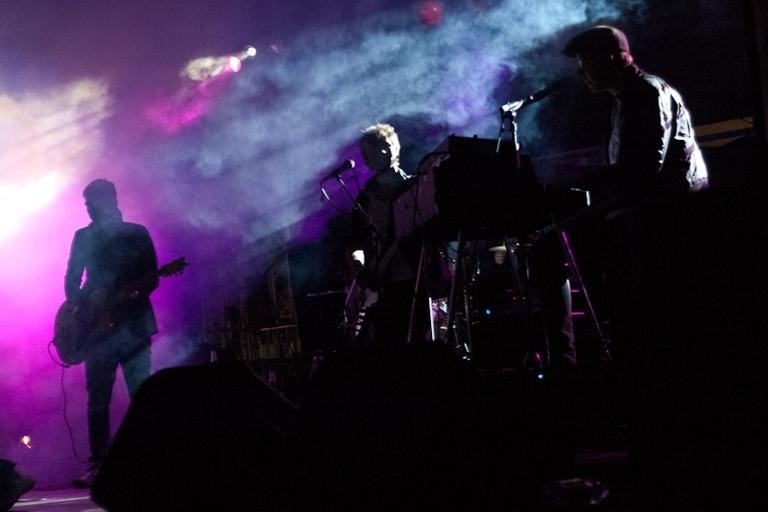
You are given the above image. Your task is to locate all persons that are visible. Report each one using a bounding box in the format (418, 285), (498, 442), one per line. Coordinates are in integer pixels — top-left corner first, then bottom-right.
(351, 123), (410, 234)
(563, 25), (710, 212)
(63, 179), (159, 487)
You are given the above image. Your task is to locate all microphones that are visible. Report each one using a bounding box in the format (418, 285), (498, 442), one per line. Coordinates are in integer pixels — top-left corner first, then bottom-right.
(524, 80), (562, 104)
(320, 159), (355, 183)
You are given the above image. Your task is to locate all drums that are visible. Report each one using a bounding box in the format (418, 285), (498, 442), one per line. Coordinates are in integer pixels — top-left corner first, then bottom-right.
(439, 239), (529, 293)
(429, 296), (549, 376)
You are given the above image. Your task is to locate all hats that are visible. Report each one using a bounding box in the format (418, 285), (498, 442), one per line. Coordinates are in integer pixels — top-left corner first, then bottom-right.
(561, 26), (631, 56)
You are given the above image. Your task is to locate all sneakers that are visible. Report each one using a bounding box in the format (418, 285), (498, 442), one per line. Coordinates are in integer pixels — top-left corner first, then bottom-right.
(71, 465), (99, 488)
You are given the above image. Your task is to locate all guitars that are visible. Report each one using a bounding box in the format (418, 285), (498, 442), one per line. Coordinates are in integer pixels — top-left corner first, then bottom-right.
(54, 257), (189, 365)
(337, 236), (403, 338)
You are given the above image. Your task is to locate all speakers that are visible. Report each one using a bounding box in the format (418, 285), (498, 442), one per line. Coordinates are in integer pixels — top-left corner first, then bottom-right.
(90, 359), (301, 512)
